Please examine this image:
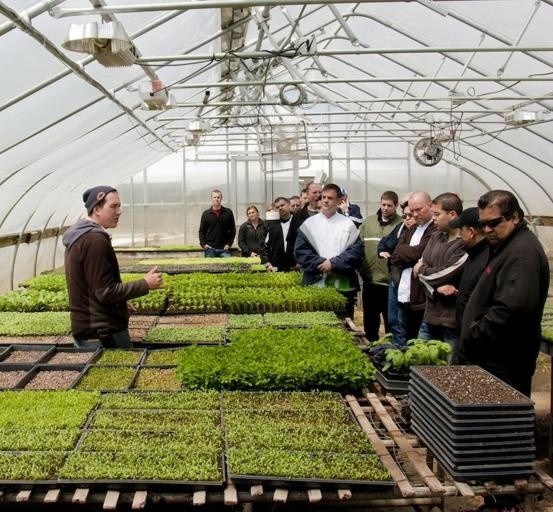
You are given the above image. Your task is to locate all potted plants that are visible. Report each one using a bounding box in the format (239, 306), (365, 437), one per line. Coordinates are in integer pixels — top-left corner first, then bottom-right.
(0, 230), (552, 501)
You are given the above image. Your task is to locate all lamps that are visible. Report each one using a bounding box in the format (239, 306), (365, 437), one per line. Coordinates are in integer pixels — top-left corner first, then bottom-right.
(503, 98), (546, 127)
(55, 8), (138, 56)
(184, 118), (213, 136)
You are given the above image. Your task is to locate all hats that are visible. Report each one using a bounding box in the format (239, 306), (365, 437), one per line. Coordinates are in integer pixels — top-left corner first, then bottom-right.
(82, 185), (117, 216)
(448, 206), (482, 229)
(336, 188), (347, 196)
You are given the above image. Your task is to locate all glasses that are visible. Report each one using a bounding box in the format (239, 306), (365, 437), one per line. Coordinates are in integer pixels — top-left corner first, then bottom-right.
(402, 213), (413, 219)
(478, 217), (504, 228)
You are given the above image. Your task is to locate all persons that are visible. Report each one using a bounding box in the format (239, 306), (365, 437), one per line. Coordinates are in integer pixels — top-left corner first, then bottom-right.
(60, 186), (161, 345)
(460, 189), (548, 397)
(198, 190), (236, 257)
